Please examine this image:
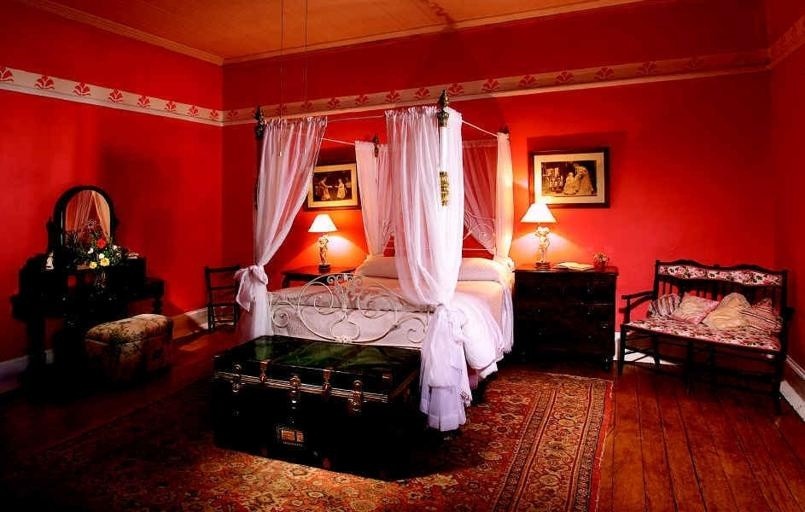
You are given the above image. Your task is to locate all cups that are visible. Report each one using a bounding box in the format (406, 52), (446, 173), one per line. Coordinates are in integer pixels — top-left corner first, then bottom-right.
(593, 259), (606, 272)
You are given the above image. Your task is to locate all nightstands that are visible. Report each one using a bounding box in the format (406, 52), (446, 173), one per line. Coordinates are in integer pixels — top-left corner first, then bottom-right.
(283, 266), (352, 286)
(512, 266), (619, 373)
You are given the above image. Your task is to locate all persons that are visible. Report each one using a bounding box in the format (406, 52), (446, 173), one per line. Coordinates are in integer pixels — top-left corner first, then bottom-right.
(319, 178), (333, 201)
(574, 164), (593, 195)
(334, 180), (347, 199)
(562, 173), (579, 195)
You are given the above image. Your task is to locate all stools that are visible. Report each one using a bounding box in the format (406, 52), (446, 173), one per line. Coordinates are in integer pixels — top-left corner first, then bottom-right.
(212, 333), (421, 466)
(86, 313), (173, 389)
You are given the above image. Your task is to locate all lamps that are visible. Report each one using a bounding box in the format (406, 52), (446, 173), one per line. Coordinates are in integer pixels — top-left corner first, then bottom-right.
(520, 203), (555, 267)
(308, 214), (336, 273)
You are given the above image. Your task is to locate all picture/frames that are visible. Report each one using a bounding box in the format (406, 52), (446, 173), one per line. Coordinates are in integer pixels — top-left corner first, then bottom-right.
(526, 148), (608, 209)
(305, 163), (361, 210)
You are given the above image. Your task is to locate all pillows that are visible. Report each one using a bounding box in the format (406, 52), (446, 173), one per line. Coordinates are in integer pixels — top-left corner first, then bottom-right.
(458, 257), (511, 285)
(355, 251), (399, 278)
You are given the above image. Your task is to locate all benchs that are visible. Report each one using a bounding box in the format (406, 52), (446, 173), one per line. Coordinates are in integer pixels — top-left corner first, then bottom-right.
(624, 258), (786, 413)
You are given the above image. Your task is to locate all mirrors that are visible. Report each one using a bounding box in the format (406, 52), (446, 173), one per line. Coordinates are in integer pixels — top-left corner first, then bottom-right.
(48, 186), (116, 259)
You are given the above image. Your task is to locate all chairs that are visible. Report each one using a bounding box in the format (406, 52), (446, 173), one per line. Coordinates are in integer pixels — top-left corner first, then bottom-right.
(203, 265), (240, 333)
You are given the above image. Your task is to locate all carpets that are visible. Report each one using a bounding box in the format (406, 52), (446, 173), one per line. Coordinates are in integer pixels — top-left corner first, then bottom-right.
(14, 367), (612, 512)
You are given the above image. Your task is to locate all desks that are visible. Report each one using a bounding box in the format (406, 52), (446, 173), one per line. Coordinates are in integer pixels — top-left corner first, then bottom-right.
(9, 253), (163, 396)
